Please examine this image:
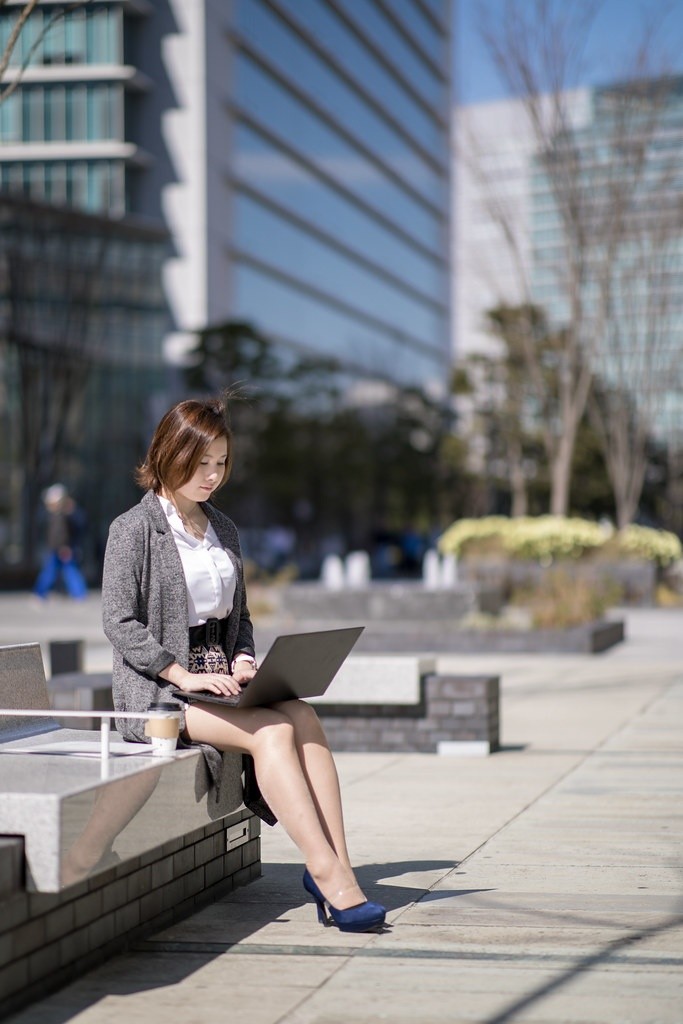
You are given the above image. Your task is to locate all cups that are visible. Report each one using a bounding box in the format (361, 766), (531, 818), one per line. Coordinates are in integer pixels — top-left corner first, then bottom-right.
(147, 703), (182, 757)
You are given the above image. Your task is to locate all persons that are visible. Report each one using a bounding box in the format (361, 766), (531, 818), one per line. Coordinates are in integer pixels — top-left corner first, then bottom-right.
(34, 483), (89, 599)
(102, 400), (385, 932)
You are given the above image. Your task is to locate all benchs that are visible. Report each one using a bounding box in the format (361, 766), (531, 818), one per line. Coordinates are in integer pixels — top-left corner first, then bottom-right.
(47, 639), (504, 755)
(0, 641), (262, 1023)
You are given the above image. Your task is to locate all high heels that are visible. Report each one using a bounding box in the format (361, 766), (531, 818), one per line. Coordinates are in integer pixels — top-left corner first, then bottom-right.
(303, 867), (386, 931)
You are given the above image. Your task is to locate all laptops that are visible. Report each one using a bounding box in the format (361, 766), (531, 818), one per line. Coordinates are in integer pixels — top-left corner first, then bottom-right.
(169, 626), (365, 707)
